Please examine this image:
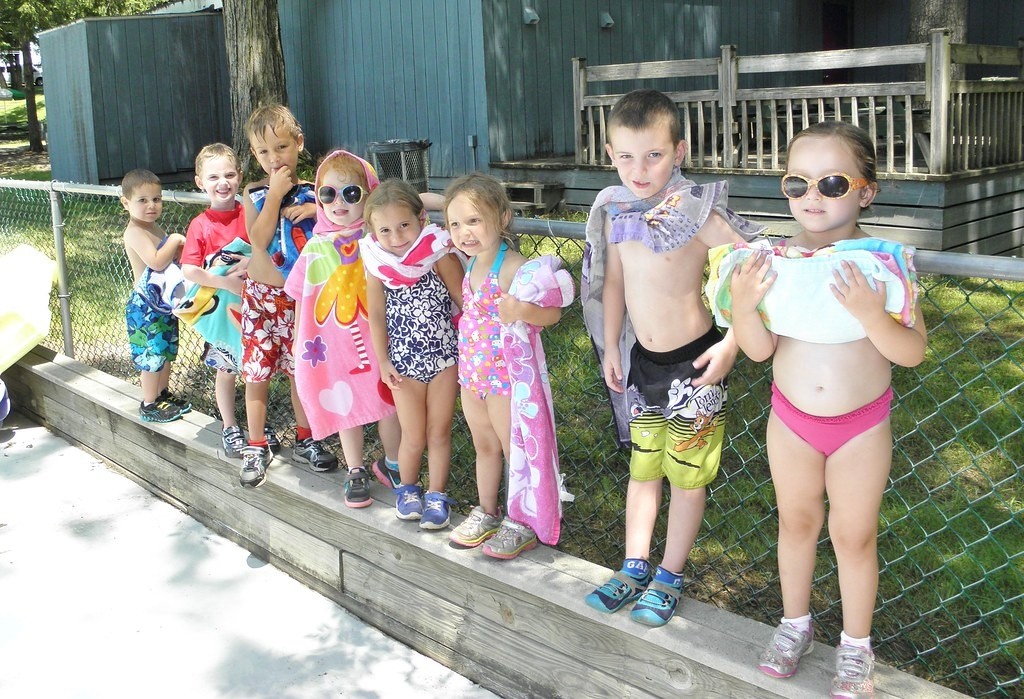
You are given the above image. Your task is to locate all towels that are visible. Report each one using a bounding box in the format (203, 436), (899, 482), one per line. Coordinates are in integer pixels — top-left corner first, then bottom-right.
(249, 185), (317, 271)
(171, 237), (251, 364)
(702, 235), (917, 346)
(133, 231), (171, 316)
(578, 168), (765, 443)
(360, 226), (472, 292)
(502, 250), (579, 546)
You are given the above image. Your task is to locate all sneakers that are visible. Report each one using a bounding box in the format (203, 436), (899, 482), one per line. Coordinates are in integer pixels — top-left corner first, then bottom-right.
(419, 491), (457, 529)
(757, 619), (814, 678)
(292, 433), (338, 471)
(482, 515), (537, 560)
(631, 565), (685, 627)
(393, 484), (423, 520)
(373, 454), (401, 491)
(238, 443), (274, 489)
(161, 388), (192, 414)
(830, 642), (876, 699)
(585, 558), (653, 614)
(221, 420), (244, 458)
(264, 424), (281, 452)
(450, 505), (504, 548)
(343, 467), (373, 508)
(138, 395), (183, 423)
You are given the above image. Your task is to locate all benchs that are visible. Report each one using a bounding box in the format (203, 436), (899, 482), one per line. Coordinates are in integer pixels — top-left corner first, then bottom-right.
(718, 112), (931, 168)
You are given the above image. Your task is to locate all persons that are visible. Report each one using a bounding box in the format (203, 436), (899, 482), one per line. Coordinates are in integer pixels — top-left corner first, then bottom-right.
(180, 142), (280, 459)
(581, 89), (767, 630)
(283, 147), (449, 507)
(357, 177), (464, 531)
(120, 168), (193, 422)
(442, 174), (562, 561)
(728, 121), (928, 699)
(240, 94), (339, 489)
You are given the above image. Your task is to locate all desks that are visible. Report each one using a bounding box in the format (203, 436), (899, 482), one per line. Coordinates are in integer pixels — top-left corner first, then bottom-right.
(761, 95), (928, 158)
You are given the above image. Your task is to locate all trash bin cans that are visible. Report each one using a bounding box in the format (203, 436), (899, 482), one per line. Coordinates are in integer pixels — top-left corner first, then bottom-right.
(366, 137), (430, 194)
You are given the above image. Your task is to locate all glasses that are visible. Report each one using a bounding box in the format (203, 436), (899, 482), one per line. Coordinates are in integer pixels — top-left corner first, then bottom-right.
(317, 184), (369, 205)
(780, 173), (868, 201)
